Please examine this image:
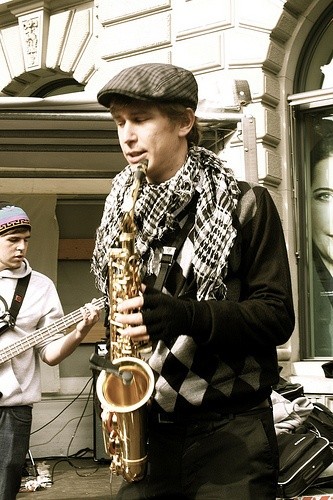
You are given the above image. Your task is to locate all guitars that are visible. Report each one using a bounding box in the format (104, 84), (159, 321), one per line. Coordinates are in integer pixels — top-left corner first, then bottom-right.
(0, 296), (108, 365)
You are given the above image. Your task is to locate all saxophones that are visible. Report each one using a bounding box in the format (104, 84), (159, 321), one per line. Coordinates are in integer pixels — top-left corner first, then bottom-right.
(95, 159), (157, 485)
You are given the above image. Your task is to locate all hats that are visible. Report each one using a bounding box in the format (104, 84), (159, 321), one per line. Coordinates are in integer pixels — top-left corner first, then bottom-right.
(96, 63), (198, 110)
(0, 206), (30, 235)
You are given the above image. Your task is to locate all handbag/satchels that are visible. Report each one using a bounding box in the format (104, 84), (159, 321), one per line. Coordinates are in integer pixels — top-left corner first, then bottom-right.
(273, 431), (332, 499)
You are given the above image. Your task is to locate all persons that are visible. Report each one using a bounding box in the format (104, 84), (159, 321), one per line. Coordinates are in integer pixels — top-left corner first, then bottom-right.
(0, 206), (102, 500)
(311, 132), (333, 357)
(91, 63), (296, 500)
